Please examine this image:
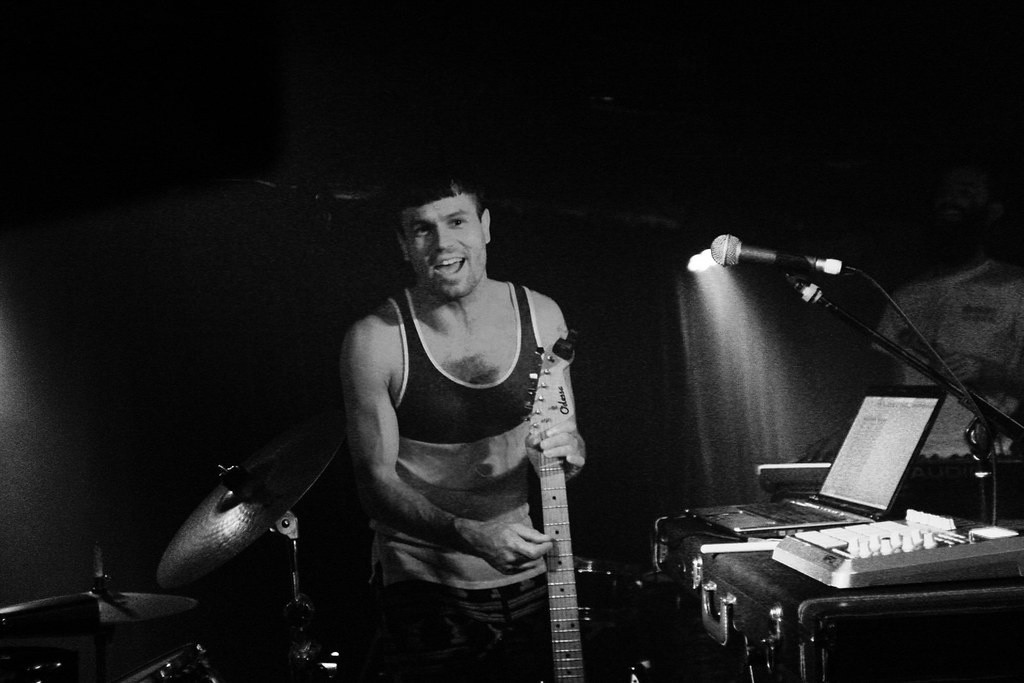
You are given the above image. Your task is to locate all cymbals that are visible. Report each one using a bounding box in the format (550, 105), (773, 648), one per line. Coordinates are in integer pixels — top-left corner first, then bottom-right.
(155, 409), (348, 589)
(0, 588), (198, 626)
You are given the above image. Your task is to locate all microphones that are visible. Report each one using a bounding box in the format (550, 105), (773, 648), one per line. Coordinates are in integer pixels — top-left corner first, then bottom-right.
(710, 235), (863, 276)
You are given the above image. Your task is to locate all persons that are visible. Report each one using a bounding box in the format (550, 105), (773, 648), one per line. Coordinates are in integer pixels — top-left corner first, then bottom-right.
(340, 163), (642, 683)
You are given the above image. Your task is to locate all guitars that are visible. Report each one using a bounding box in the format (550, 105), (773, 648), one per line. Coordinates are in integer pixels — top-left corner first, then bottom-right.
(524, 338), (585, 681)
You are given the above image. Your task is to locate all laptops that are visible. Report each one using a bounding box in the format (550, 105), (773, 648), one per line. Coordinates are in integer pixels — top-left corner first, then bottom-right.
(691, 384), (948, 537)
